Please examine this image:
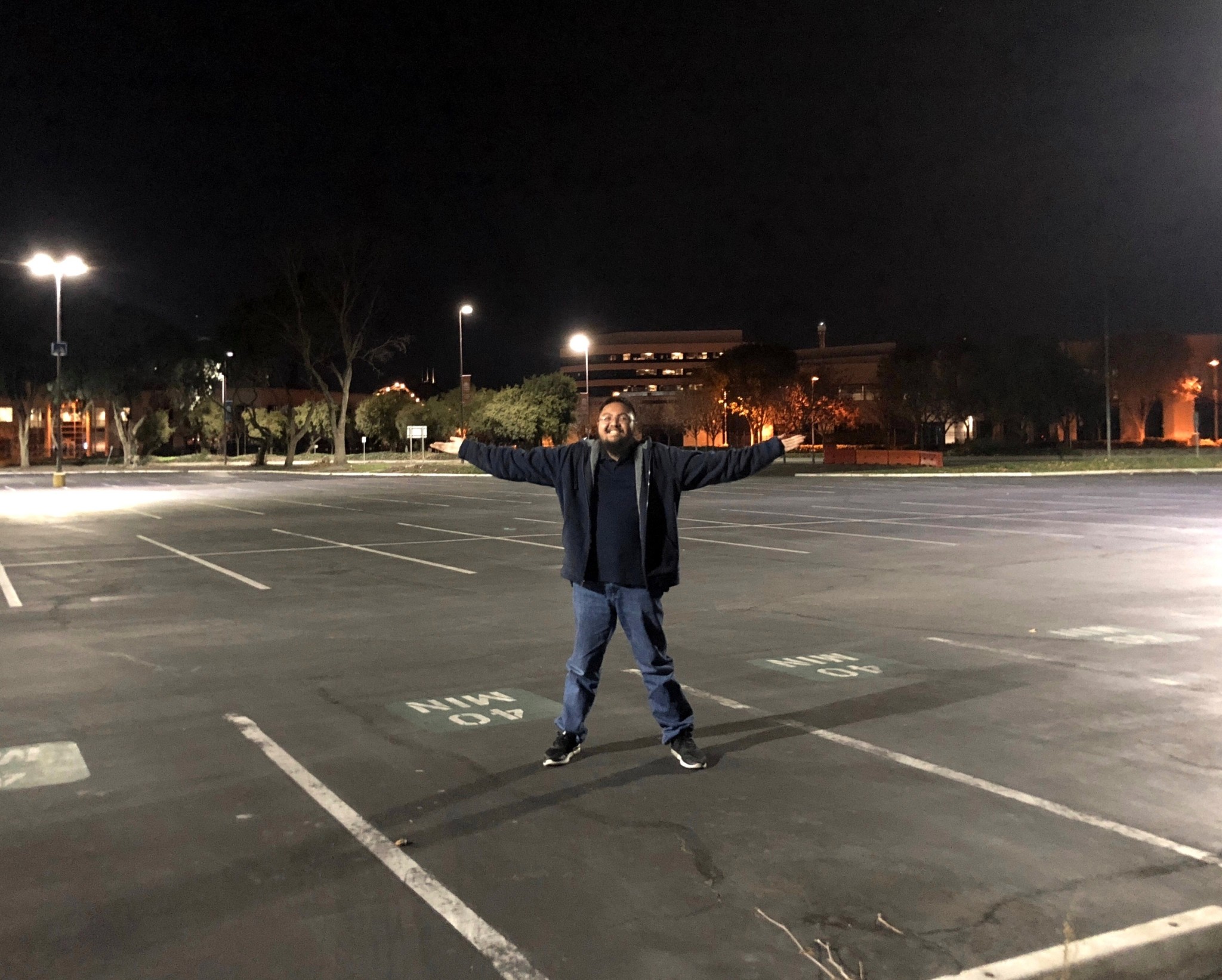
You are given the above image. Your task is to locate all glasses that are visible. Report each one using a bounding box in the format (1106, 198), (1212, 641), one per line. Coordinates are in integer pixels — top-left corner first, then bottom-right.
(599, 415), (635, 424)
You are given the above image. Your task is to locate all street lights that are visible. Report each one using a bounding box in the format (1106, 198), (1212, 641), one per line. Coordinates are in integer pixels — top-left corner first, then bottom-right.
(811, 376), (819, 464)
(222, 350), (234, 465)
(569, 334), (591, 442)
(459, 305), (473, 463)
(24, 252), (88, 487)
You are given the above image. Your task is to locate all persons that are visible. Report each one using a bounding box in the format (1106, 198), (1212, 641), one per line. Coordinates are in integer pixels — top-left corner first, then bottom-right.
(427, 396), (806, 770)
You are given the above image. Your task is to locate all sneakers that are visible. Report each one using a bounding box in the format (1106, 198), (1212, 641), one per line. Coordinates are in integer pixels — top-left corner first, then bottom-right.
(671, 733), (707, 768)
(543, 731), (581, 766)
